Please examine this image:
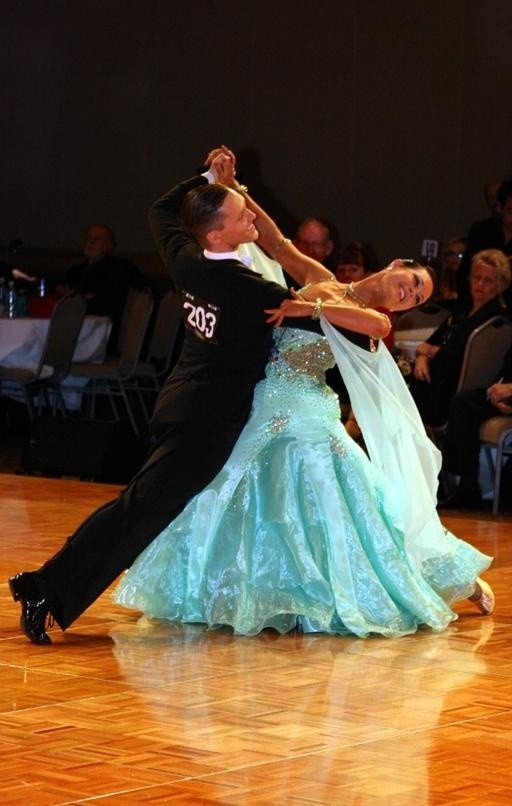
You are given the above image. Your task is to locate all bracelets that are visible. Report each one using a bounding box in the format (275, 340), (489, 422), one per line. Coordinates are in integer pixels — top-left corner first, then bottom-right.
(235, 184), (249, 194)
(311, 298), (325, 321)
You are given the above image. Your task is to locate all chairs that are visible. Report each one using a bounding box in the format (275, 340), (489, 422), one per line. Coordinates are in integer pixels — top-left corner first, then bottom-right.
(395, 303), (510, 510)
(0, 286), (185, 441)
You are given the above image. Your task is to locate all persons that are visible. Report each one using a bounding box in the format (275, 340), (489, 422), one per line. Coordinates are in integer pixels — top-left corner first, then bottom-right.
(6, 144), (379, 647)
(110, 142), (495, 638)
(291, 171), (511, 512)
(43, 224), (128, 361)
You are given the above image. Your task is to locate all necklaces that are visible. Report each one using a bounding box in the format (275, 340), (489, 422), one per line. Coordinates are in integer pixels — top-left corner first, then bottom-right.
(345, 280), (369, 308)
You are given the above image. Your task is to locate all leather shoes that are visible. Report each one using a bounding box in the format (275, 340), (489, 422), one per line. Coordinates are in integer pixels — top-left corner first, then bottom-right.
(473, 576), (494, 615)
(9, 572), (51, 646)
(438, 489), (483, 509)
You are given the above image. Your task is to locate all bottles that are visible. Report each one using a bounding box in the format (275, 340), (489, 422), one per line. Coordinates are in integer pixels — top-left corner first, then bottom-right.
(0, 277), (16, 305)
(36, 275), (46, 299)
